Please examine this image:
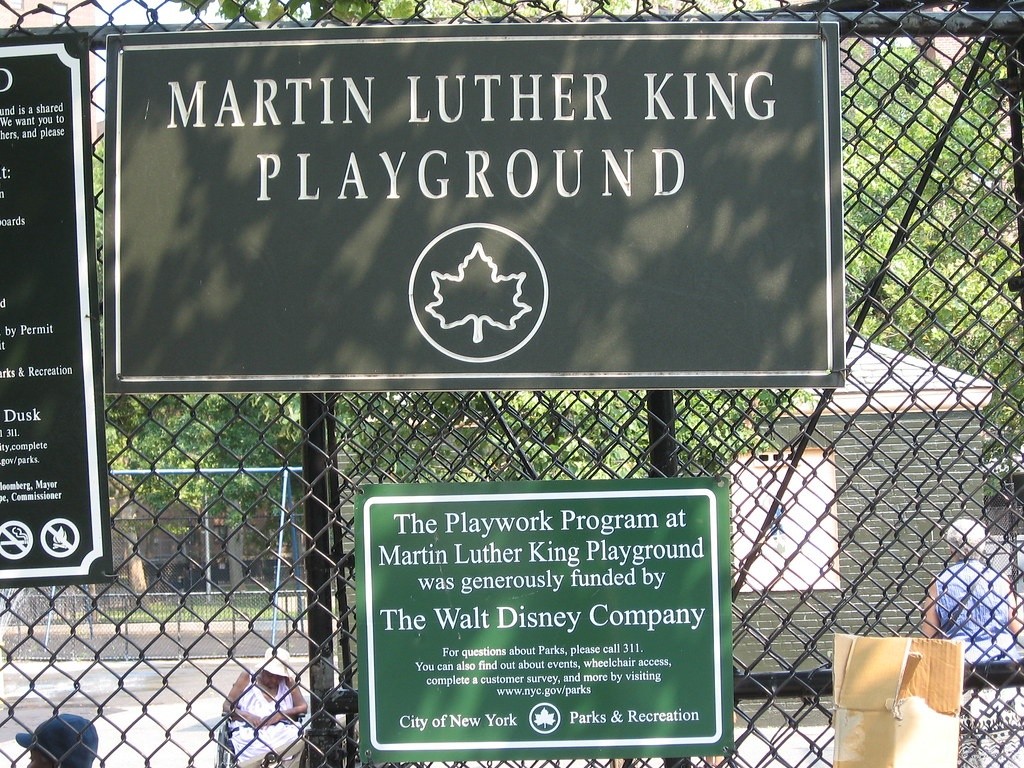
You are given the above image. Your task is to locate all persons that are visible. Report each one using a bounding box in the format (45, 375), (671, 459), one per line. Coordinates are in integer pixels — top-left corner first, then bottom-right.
(13, 714), (98, 768)
(921, 519), (1023, 768)
(610, 756), (726, 768)
(222, 646), (309, 768)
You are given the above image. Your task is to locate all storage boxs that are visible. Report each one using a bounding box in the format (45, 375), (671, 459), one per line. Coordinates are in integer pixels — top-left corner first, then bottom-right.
(832, 633), (965, 768)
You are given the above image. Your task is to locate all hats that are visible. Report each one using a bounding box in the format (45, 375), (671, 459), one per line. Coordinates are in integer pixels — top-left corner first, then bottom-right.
(15, 714), (98, 768)
(264, 647), (289, 677)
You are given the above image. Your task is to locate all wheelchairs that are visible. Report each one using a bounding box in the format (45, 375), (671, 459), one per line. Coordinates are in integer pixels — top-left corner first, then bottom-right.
(214, 711), (306, 768)
